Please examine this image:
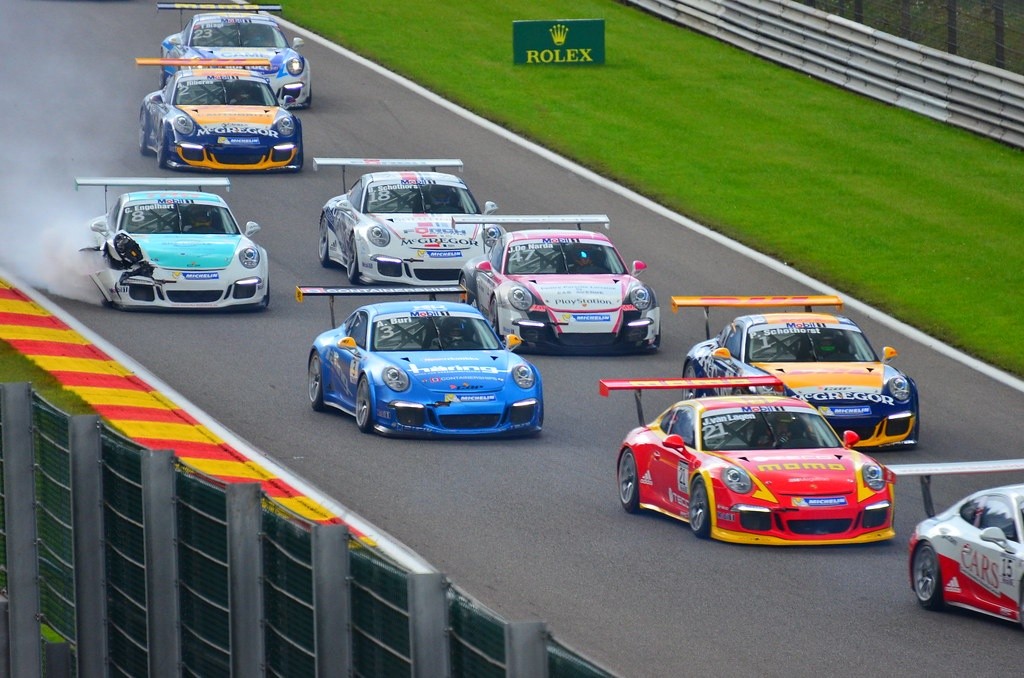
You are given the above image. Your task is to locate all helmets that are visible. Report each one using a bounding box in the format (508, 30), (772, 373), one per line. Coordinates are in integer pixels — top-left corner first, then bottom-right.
(427, 184), (452, 205)
(774, 413), (792, 422)
(814, 331), (837, 354)
(187, 204), (212, 226)
(438, 317), (467, 345)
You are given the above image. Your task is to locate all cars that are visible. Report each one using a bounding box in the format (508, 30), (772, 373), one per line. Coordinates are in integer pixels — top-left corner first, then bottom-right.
(154, 2), (314, 112)
(670, 290), (919, 450)
(450, 210), (664, 354)
(310, 155), (514, 291)
(293, 278), (543, 439)
(73, 174), (273, 313)
(131, 49), (302, 175)
(885, 456), (1024, 628)
(600, 375), (894, 549)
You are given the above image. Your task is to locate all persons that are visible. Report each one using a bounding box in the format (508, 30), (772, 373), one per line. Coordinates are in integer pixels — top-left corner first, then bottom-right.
(229, 84), (261, 105)
(174, 206), (220, 234)
(563, 244), (603, 274)
(429, 319), (469, 351)
(796, 331), (848, 362)
(758, 413), (810, 450)
